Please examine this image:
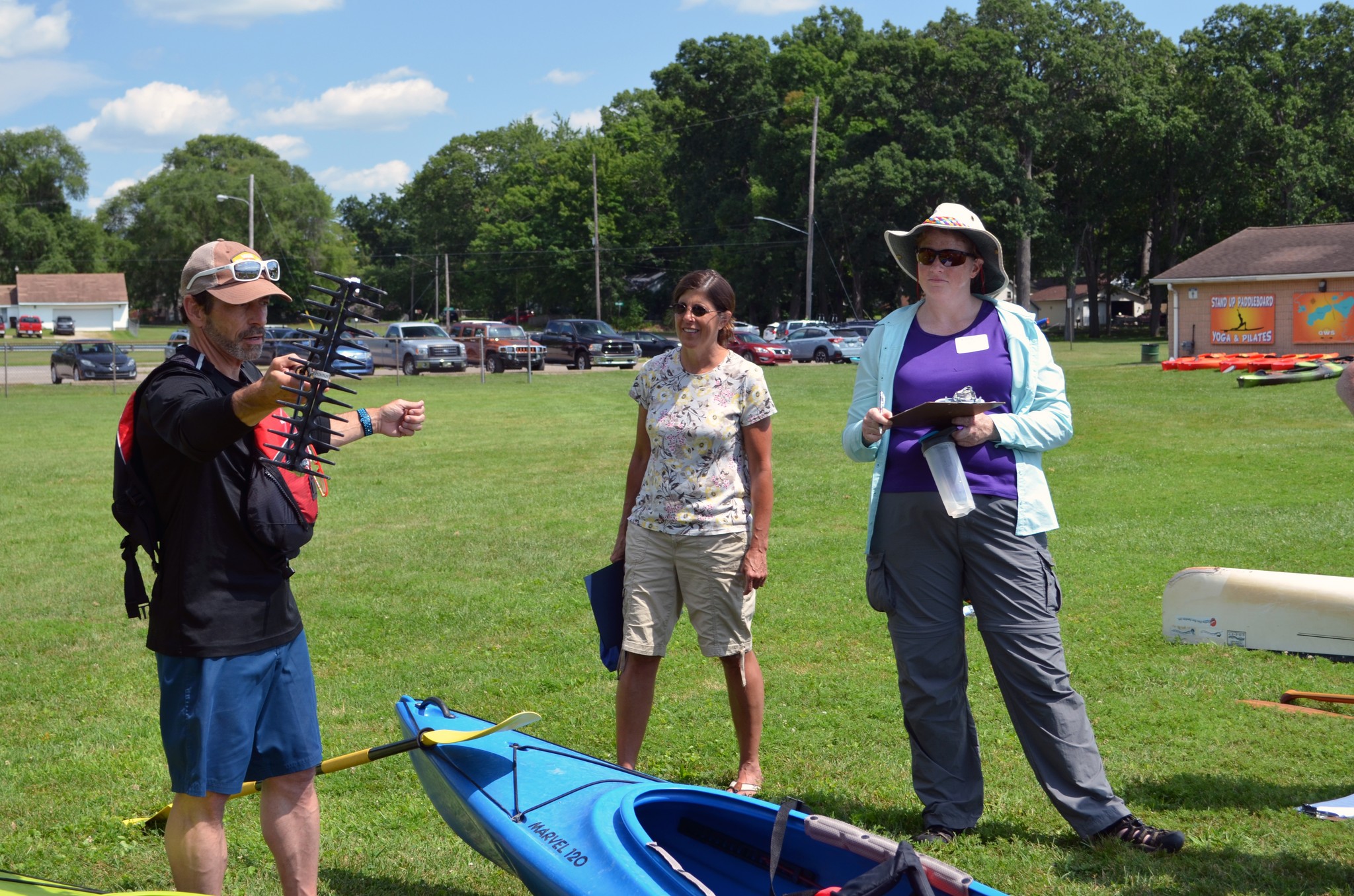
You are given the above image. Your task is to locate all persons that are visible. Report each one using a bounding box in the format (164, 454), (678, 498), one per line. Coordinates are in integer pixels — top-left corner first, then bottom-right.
(110, 238), (425, 896)
(840, 202), (1185, 857)
(610, 269), (778, 798)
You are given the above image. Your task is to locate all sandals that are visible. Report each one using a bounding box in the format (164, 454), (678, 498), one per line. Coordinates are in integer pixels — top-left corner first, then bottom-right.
(912, 825), (957, 845)
(1090, 813), (1184, 855)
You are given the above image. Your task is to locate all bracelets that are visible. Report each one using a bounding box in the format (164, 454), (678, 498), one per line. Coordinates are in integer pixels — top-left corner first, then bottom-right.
(357, 408), (373, 437)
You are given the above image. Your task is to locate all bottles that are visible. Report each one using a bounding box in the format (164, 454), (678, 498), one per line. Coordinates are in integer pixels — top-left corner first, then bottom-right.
(919, 427), (978, 520)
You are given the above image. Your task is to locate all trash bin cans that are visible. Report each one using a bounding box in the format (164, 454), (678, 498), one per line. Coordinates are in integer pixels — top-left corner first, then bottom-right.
(1141, 343), (1160, 362)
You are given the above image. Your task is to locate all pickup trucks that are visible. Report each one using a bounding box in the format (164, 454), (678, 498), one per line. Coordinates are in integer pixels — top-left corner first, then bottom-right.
(528, 318), (642, 372)
(363, 322), (472, 374)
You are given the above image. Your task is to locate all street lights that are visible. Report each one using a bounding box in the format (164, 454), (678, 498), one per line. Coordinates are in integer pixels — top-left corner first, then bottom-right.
(216, 194), (255, 250)
(754, 216), (813, 320)
(394, 252), (441, 323)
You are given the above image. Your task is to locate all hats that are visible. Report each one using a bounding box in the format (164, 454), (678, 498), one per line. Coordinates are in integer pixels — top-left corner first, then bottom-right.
(884, 202), (1009, 298)
(180, 238), (293, 306)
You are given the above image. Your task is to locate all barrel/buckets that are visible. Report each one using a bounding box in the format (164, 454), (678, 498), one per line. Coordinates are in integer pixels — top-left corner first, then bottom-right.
(1141, 345), (1159, 362)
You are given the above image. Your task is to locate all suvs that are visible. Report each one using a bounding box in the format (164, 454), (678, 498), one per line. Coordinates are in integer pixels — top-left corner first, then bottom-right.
(449, 320), (548, 371)
(164, 329), (195, 356)
(14, 315), (43, 338)
(53, 316), (76, 336)
(767, 325), (867, 361)
(763, 319), (878, 342)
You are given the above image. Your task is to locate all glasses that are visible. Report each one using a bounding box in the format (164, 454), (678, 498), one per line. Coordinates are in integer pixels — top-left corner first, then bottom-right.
(672, 303), (720, 318)
(914, 246), (968, 268)
(184, 259), (280, 290)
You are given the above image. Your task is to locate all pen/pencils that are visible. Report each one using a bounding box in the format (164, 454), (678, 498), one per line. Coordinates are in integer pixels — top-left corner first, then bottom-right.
(879, 391), (885, 434)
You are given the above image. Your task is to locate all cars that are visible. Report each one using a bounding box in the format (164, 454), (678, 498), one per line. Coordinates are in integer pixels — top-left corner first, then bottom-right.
(729, 322), (763, 333)
(49, 339), (138, 384)
(438, 310), (460, 322)
(727, 331), (794, 362)
(278, 329), (378, 368)
(253, 326), (295, 365)
(0, 316), (6, 335)
(618, 330), (683, 355)
(502, 310), (542, 322)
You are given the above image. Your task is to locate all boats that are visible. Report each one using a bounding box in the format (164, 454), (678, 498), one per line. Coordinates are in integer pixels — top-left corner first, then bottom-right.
(1160, 350), (1345, 388)
(1161, 565), (1354, 659)
(394, 695), (1009, 894)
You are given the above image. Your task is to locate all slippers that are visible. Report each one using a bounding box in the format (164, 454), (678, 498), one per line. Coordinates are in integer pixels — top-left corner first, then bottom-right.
(726, 781), (763, 798)
